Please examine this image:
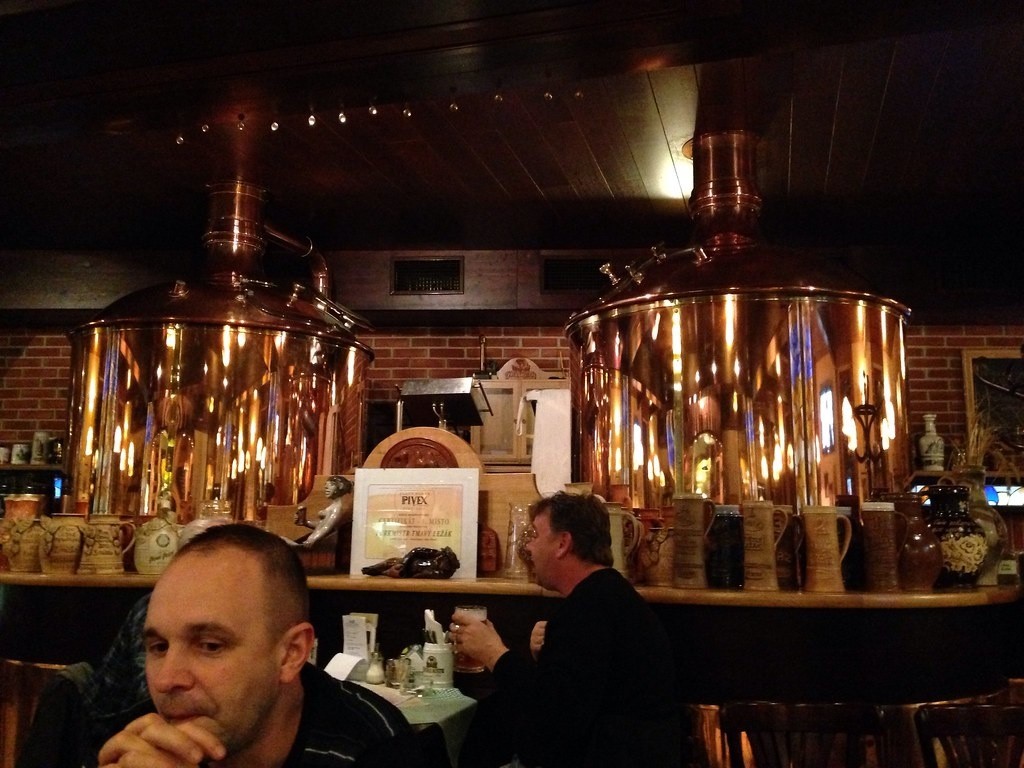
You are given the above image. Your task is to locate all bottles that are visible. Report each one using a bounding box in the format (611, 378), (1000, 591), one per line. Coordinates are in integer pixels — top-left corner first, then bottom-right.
(134, 501), (180, 574)
(925, 485), (987, 594)
(703, 504), (743, 590)
(639, 528), (676, 587)
(949, 464), (1007, 587)
(919, 414), (945, 471)
(366, 643), (421, 695)
(880, 495), (943, 594)
(178, 499), (234, 550)
(1, 493), (85, 575)
(477, 490), (500, 578)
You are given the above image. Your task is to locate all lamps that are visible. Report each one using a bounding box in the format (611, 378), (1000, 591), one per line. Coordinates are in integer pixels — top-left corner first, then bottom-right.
(495, 90), (504, 103)
(176, 132), (185, 145)
(402, 103), (413, 118)
(575, 86), (585, 100)
(369, 103), (379, 117)
(543, 88), (554, 101)
(448, 99), (467, 114)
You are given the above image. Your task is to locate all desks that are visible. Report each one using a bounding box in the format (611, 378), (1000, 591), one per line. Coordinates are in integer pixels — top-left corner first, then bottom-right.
(368, 678), (525, 768)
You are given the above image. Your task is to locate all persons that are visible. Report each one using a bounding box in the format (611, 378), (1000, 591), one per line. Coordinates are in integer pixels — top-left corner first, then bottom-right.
(445, 491), (696, 768)
(79, 524), (431, 768)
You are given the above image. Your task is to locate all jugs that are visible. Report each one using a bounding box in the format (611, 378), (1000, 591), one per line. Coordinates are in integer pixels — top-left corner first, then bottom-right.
(77, 514), (137, 574)
(504, 501), (537, 580)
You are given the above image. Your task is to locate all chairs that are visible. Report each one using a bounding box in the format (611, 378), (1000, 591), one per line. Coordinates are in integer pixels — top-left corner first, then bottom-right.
(718, 702), (889, 768)
(913, 705), (1024, 768)
(0, 656), (93, 768)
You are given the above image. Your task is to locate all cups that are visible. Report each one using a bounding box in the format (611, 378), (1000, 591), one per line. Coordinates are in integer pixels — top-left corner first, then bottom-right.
(0, 432), (65, 465)
(565, 481), (910, 594)
(423, 642), (453, 689)
(453, 606), (486, 672)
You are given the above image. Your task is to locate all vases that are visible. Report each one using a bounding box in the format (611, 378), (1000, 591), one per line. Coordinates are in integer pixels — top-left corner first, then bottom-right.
(920, 414), (945, 472)
(949, 464), (1008, 585)
(0, 493), (45, 573)
(885, 493), (944, 594)
(39, 513), (86, 574)
(925, 485), (988, 591)
(29, 432), (51, 465)
(11, 444), (29, 465)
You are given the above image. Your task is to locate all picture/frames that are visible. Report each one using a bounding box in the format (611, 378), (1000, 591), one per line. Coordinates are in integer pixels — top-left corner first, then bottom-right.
(962, 346), (1024, 473)
